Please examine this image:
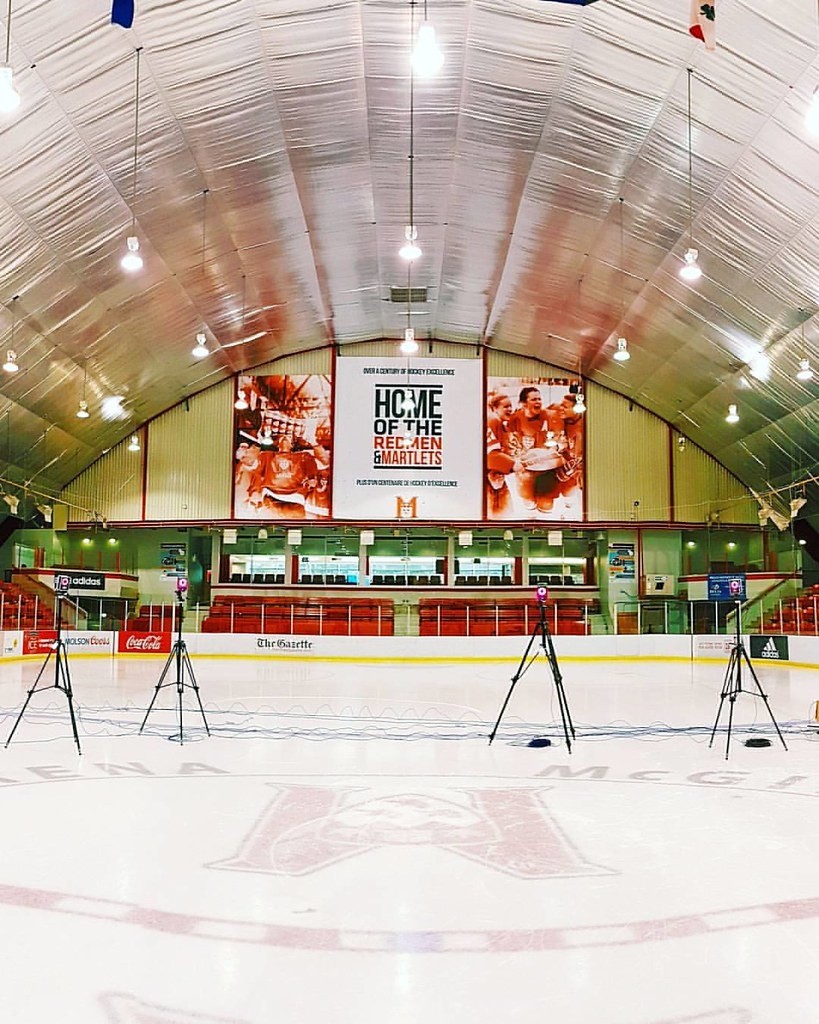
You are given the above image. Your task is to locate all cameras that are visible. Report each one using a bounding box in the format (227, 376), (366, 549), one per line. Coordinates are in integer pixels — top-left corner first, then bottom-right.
(56, 575), (71, 591)
(177, 577), (188, 591)
(537, 585), (547, 601)
(729, 579), (740, 595)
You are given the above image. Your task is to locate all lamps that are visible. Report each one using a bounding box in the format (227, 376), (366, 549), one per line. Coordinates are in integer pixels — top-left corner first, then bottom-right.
(678, 425), (687, 454)
(3, 298), (20, 375)
(399, 2), (421, 262)
(796, 310), (812, 381)
(127, 418), (138, 454)
(414, 1), (437, 50)
(107, 525), (117, 545)
(686, 530), (695, 548)
(612, 203), (631, 364)
(0, 1), (15, 112)
(77, 363), (90, 421)
(726, 529), (736, 550)
(725, 371), (738, 426)
(678, 71), (702, 284)
(399, 264), (419, 352)
(573, 356), (586, 415)
(119, 50), (144, 272)
(83, 525), (94, 546)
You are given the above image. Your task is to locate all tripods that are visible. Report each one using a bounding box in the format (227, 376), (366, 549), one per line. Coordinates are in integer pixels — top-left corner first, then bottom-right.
(488, 602), (575, 755)
(137, 592), (210, 746)
(4, 593), (82, 756)
(710, 600), (788, 761)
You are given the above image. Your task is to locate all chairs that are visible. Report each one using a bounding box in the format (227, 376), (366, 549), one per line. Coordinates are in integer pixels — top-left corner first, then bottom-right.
(229, 576), (573, 586)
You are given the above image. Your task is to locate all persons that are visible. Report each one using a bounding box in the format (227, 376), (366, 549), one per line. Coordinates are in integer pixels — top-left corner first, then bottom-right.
(302, 442), (333, 519)
(541, 390), (583, 513)
(505, 385), (570, 514)
(486, 393), (525, 521)
(257, 432), (313, 519)
(235, 440), (270, 519)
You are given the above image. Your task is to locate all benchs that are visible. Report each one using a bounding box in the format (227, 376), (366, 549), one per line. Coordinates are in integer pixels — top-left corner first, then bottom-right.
(0, 580), (76, 630)
(760, 585), (819, 635)
(122, 605), (183, 632)
(710, 562), (740, 573)
(201, 596), (393, 636)
(420, 597), (590, 635)
